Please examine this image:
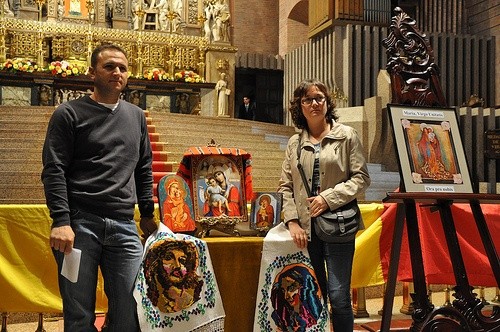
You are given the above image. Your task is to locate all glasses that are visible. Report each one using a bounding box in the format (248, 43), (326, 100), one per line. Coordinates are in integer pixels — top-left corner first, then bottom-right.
(301, 96), (327, 105)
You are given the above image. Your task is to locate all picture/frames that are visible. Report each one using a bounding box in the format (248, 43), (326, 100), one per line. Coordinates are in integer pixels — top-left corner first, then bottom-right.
(191, 155), (248, 222)
(387, 103), (476, 194)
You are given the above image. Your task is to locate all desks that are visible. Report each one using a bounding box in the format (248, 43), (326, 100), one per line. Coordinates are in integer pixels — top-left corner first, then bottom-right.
(136, 215), (311, 332)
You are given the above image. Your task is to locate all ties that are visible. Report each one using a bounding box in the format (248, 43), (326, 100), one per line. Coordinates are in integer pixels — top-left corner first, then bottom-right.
(246, 105), (248, 112)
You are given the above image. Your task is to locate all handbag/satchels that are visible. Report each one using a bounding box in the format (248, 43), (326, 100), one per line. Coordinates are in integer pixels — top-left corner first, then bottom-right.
(314, 198), (360, 243)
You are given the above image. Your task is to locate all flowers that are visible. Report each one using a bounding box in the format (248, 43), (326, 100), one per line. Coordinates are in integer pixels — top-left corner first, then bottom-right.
(175, 71), (207, 83)
(143, 68), (174, 81)
(49, 61), (78, 77)
(3, 58), (41, 73)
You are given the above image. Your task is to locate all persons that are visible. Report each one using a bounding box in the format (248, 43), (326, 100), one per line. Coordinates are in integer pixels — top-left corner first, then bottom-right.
(37, 85), (190, 114)
(41, 44), (157, 332)
(41, 2), (47, 22)
(278, 80), (371, 332)
(56, 0), (65, 22)
(215, 73), (231, 117)
(85, 0), (96, 25)
(237, 97), (256, 121)
(105, 2), (114, 29)
(130, 0), (230, 42)
